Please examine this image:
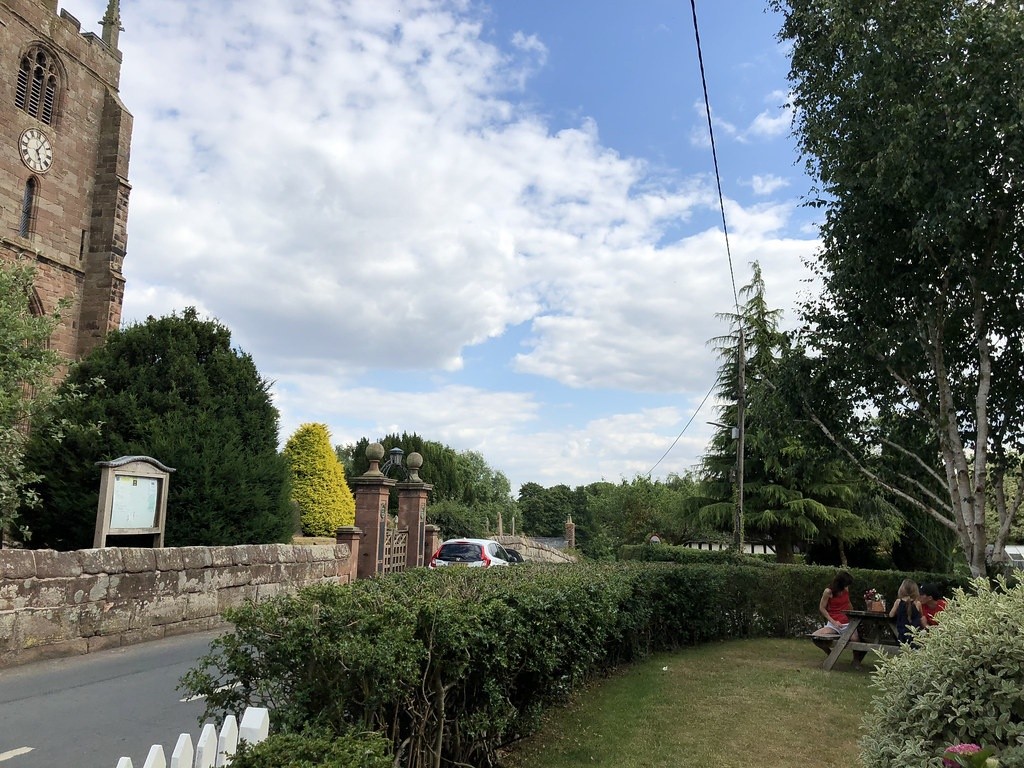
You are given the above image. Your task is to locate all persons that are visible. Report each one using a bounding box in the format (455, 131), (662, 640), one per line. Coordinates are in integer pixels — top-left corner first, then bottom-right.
(812, 572), (867, 672)
(889, 578), (947, 649)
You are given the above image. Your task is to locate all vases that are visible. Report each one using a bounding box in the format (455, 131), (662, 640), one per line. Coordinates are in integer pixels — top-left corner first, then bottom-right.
(865, 600), (886, 612)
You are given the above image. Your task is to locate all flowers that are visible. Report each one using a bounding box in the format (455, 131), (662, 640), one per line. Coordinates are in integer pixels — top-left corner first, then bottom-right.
(864, 588), (884, 602)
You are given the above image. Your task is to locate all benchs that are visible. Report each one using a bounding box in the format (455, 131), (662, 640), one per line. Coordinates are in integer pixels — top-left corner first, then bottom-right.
(806, 633), (841, 641)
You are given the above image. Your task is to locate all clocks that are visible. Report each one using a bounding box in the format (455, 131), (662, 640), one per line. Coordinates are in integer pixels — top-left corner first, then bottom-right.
(19, 127), (54, 173)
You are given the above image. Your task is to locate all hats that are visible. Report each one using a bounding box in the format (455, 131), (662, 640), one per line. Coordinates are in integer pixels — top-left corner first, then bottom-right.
(918, 582), (939, 592)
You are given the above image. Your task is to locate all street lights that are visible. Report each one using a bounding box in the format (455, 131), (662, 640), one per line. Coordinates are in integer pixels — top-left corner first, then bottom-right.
(705, 419), (744, 552)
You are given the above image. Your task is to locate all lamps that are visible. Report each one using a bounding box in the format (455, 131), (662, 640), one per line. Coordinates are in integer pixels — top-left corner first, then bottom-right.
(390, 447), (404, 466)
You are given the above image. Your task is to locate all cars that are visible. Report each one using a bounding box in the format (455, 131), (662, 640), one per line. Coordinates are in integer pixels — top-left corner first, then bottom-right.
(428, 538), (511, 570)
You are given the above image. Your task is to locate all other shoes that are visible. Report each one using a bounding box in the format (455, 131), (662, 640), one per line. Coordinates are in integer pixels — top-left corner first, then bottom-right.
(851, 659), (863, 672)
(832, 661), (840, 670)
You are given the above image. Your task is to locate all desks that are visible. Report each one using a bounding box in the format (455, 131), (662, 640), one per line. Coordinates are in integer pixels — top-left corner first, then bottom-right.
(816, 610), (901, 672)
